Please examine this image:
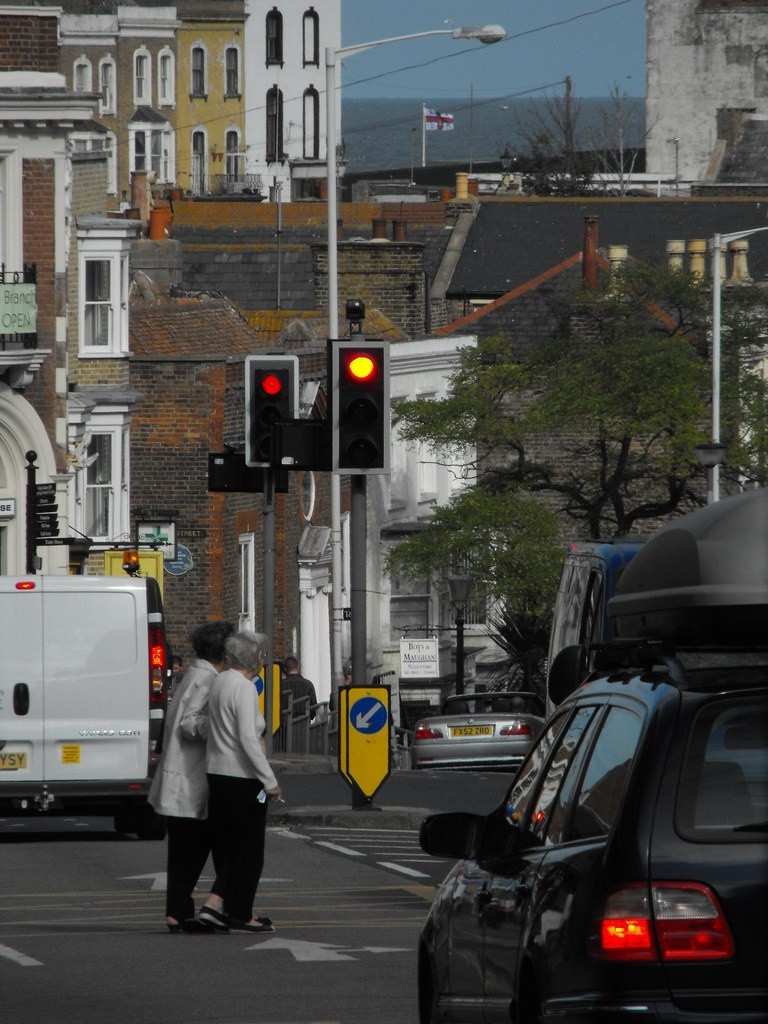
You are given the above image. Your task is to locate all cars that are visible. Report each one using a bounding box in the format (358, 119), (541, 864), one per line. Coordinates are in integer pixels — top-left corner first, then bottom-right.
(410, 689), (546, 773)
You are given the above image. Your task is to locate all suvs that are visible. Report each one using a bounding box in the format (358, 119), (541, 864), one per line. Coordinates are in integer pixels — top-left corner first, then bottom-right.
(415, 489), (767, 1024)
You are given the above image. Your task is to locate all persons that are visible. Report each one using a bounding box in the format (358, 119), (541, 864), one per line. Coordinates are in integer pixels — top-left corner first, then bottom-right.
(343, 664), (354, 686)
(147, 620), (235, 933)
(170, 654), (184, 698)
(280, 657), (318, 723)
(198, 629), (281, 934)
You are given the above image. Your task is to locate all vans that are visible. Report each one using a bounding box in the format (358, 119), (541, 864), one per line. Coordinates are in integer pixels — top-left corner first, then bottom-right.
(548, 533), (647, 717)
(1, 543), (174, 842)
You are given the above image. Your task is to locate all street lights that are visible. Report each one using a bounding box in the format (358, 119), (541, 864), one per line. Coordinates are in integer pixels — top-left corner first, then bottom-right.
(327, 24), (509, 808)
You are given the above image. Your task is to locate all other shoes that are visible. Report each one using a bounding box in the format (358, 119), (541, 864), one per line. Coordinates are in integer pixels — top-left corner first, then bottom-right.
(228, 917), (274, 934)
(198, 906), (227, 928)
(168, 898), (194, 931)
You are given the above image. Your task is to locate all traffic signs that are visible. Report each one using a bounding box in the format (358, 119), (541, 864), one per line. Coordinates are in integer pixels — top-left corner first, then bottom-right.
(35, 483), (76, 545)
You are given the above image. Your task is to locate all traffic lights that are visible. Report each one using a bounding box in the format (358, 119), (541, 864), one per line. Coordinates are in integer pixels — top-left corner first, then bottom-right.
(332, 340), (391, 476)
(245, 353), (300, 469)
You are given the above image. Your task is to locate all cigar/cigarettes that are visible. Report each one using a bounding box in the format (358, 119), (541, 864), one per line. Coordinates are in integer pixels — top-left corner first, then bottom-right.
(280, 798), (286, 803)
